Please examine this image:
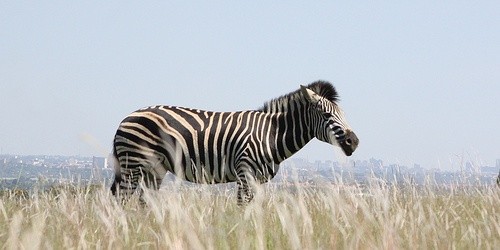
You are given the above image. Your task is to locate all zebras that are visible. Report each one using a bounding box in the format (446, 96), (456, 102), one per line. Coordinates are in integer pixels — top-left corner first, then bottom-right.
(110, 80), (360, 209)
